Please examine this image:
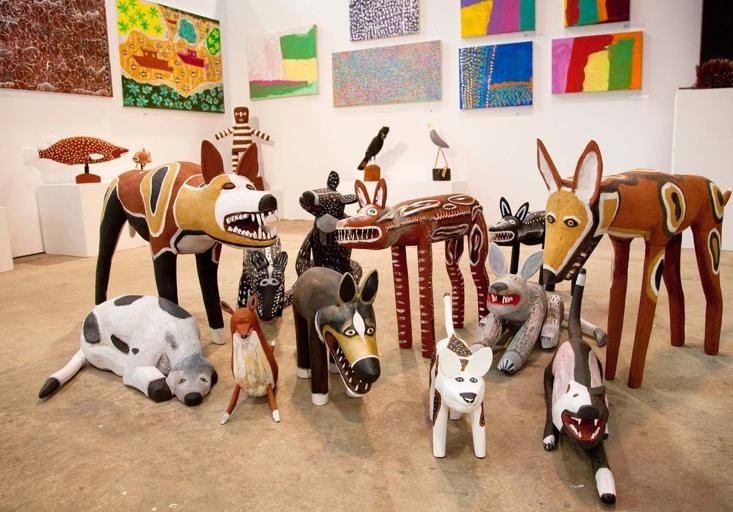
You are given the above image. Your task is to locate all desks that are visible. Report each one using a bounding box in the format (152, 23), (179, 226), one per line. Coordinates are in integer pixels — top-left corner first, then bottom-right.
(37, 183), (146, 258)
